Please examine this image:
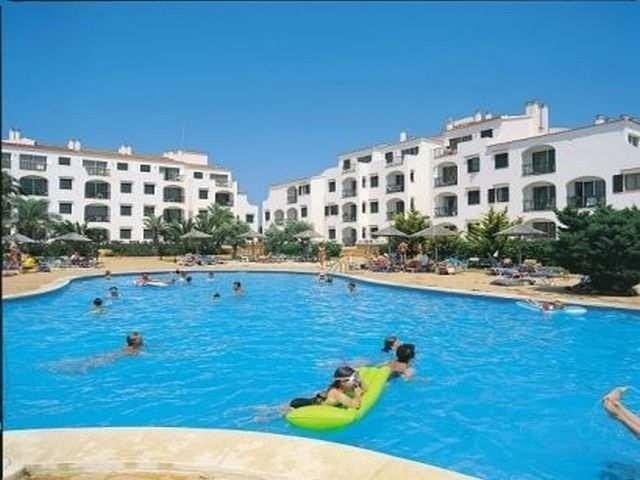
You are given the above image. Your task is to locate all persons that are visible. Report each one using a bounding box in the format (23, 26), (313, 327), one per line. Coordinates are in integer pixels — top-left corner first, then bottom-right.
(601, 385), (640, 438)
(527, 297), (554, 314)
(533, 259), (544, 273)
(210, 292), (221, 299)
(91, 297), (109, 318)
(136, 269), (193, 287)
(105, 270), (112, 280)
(44, 331), (149, 374)
(502, 255), (513, 268)
(246, 365), (368, 423)
(554, 300), (567, 310)
(3, 242), (224, 277)
(383, 336), (400, 352)
(316, 241), (436, 292)
(208, 272), (214, 279)
(232, 281), (244, 296)
(105, 286), (121, 300)
(380, 342), (418, 380)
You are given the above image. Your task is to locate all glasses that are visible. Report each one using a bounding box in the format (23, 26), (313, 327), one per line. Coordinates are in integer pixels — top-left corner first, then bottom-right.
(346, 372), (359, 383)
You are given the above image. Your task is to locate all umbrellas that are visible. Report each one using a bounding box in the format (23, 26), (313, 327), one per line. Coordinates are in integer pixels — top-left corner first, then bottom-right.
(488, 222), (549, 242)
(180, 228), (213, 253)
(369, 225), (408, 253)
(54, 232), (92, 247)
(410, 222), (457, 247)
(293, 229), (323, 244)
(1, 232), (40, 245)
(236, 229), (268, 240)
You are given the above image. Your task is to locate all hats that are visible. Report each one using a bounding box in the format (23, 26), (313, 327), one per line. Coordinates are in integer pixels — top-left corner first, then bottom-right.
(333, 366), (355, 382)
